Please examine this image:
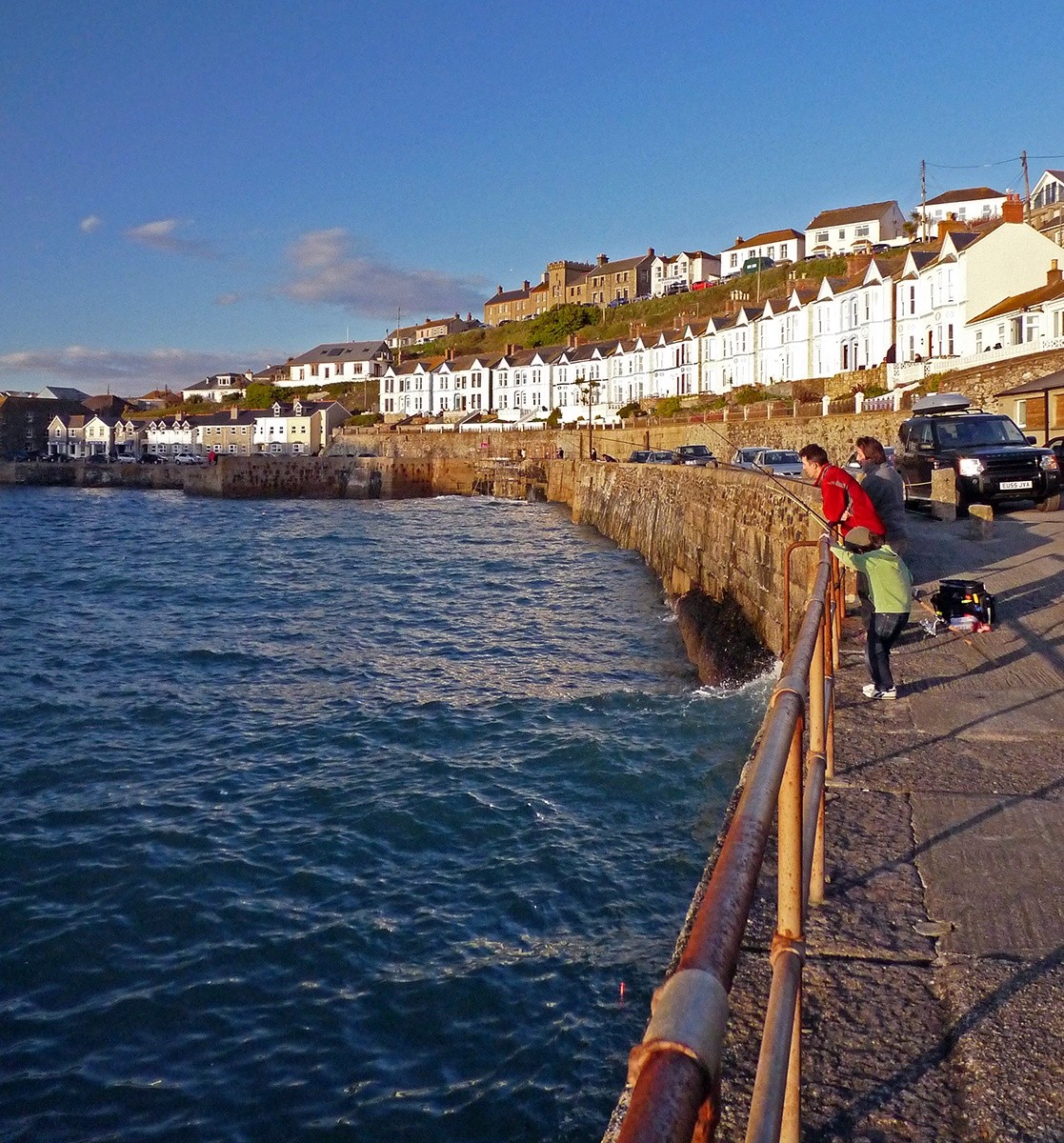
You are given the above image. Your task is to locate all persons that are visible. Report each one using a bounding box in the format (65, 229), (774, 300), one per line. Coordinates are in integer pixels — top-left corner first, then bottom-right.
(799, 444), (886, 645)
(818, 527), (914, 699)
(522, 448), (527, 461)
(851, 436), (908, 615)
(591, 448), (597, 462)
(603, 454), (617, 462)
(545, 447), (563, 459)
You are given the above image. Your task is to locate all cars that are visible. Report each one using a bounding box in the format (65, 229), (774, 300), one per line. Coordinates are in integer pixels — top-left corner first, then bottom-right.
(413, 236), (938, 347)
(751, 450), (804, 478)
(627, 450), (654, 463)
(87, 454), (116, 463)
(357, 452), (379, 457)
(840, 447), (896, 481)
(174, 452), (208, 465)
(117, 453), (140, 464)
(139, 454), (168, 465)
(250, 452), (276, 458)
(730, 447), (772, 471)
(645, 451), (673, 465)
(52, 453), (76, 463)
(1041, 435), (1064, 487)
(214, 453), (236, 461)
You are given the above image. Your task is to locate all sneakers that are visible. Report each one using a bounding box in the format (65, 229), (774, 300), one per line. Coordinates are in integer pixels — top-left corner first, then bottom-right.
(862, 684), (897, 700)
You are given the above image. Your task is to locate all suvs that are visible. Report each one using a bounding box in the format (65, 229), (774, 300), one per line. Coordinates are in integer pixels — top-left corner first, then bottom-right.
(887, 394), (1061, 520)
(671, 444), (719, 469)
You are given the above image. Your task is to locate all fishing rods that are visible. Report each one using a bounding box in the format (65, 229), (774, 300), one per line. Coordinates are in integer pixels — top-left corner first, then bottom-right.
(580, 435), (603, 457)
(667, 391), (1003, 669)
(555, 434), (593, 456)
(549, 430), (823, 489)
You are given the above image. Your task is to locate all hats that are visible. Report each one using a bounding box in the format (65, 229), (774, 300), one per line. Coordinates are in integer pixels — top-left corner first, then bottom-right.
(841, 526), (874, 551)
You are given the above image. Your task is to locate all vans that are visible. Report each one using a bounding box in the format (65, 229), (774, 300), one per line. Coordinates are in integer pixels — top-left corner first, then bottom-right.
(25, 451), (50, 462)
